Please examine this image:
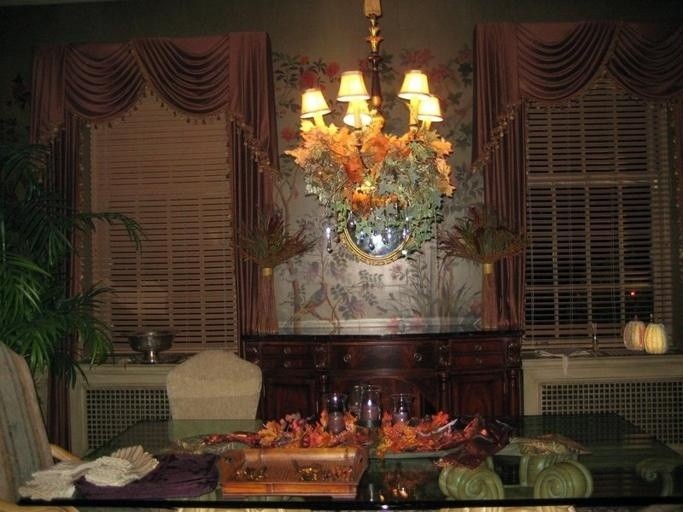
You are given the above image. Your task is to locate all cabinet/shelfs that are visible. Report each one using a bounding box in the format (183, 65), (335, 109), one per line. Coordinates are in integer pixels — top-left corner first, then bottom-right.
(321, 382), (418, 434)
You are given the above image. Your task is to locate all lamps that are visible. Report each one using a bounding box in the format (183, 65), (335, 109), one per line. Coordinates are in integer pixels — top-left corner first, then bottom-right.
(1, 340), (185, 512)
(163, 348), (265, 511)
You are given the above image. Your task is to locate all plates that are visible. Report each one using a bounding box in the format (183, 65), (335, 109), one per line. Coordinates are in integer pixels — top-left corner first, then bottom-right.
(243, 324), (525, 486)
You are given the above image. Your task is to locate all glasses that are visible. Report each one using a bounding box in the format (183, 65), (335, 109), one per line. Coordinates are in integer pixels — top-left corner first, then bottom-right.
(298, 0), (445, 133)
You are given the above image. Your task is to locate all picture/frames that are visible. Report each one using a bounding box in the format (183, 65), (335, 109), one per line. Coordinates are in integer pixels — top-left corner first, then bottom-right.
(16, 412), (682, 511)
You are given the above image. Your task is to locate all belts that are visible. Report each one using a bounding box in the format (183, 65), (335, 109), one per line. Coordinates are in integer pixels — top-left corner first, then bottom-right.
(128, 333), (179, 363)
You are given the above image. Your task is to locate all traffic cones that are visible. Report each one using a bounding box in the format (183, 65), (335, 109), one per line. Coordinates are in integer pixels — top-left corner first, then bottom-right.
(623, 315), (645, 351)
(644, 322), (669, 354)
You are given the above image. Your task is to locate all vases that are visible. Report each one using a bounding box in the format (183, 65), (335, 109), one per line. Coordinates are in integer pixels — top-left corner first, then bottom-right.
(282, 118), (459, 264)
